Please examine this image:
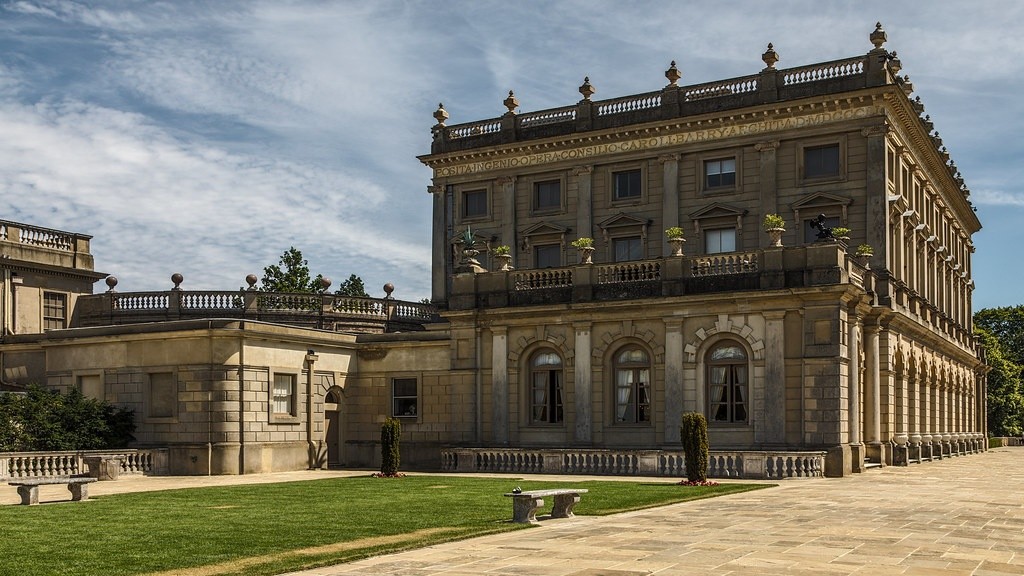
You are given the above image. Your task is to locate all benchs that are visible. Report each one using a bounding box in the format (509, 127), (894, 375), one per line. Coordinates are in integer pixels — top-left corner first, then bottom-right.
(504, 489), (589, 524)
(9, 476), (98, 505)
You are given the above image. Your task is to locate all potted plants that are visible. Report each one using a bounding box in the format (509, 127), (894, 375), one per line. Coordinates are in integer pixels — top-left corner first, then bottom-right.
(664, 227), (686, 257)
(763, 214), (785, 248)
(855, 244), (874, 270)
(832, 228), (851, 241)
(571, 237), (595, 264)
(492, 245), (512, 271)
(453, 225), (481, 266)
